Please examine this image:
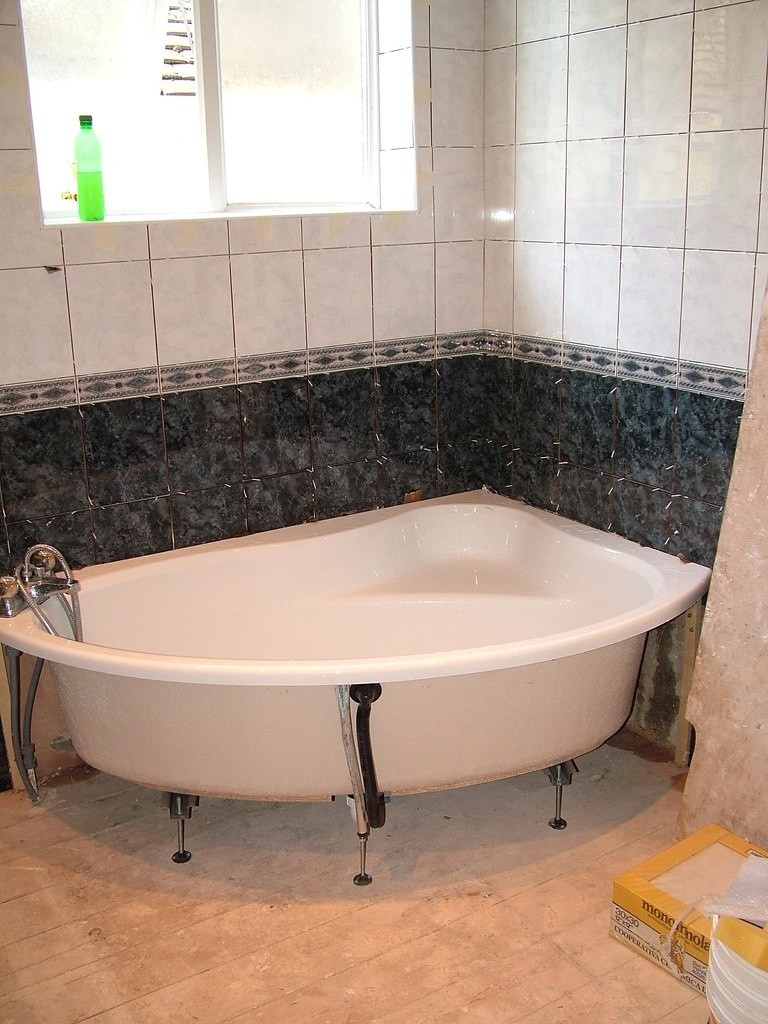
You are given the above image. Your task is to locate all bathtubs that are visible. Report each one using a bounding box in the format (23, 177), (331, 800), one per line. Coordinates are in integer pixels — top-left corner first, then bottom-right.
(5, 486), (717, 891)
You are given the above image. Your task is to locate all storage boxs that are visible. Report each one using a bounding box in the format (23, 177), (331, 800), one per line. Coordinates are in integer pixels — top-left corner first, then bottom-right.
(607, 823), (768, 997)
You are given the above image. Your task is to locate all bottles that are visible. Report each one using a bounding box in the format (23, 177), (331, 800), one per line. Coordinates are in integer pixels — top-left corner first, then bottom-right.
(74, 114), (105, 222)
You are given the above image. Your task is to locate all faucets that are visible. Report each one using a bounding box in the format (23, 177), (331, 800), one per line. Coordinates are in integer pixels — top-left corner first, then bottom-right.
(20, 563), (82, 607)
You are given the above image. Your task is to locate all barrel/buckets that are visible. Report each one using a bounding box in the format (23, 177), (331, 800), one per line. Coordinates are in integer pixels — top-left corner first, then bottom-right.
(704, 913), (768, 1024)
